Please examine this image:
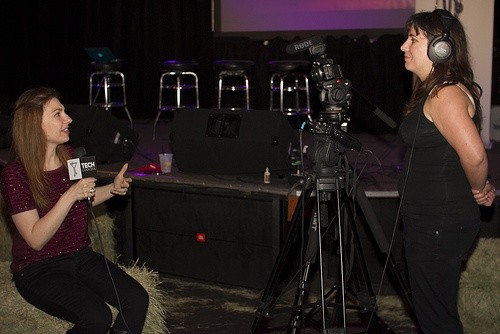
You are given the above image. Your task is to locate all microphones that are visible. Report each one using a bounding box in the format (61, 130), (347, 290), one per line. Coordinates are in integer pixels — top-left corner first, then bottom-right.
(80, 155), (97, 205)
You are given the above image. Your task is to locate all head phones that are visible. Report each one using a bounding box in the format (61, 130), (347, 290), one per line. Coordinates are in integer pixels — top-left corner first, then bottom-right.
(427, 9), (454, 63)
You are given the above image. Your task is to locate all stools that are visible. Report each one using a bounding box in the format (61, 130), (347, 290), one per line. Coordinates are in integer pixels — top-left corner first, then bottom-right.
(152, 59), (200, 140)
(213, 60), (256, 110)
(88, 60), (133, 129)
(266, 60), (313, 127)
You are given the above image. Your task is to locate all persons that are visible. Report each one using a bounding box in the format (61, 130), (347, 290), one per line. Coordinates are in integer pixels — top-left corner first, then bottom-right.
(397, 9), (496, 334)
(0, 89), (149, 334)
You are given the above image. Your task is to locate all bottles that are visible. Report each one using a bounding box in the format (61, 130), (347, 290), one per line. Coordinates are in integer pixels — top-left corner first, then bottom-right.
(264, 168), (270, 183)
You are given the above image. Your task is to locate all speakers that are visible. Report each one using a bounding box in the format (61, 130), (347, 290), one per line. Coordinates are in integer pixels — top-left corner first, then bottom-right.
(171, 108), (296, 179)
(60, 104), (129, 165)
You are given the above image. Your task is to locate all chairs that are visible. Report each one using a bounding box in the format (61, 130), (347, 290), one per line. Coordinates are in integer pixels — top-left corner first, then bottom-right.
(0, 147), (169, 334)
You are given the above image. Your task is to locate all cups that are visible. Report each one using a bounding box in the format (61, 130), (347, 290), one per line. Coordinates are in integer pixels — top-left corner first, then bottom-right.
(159, 153), (173, 173)
